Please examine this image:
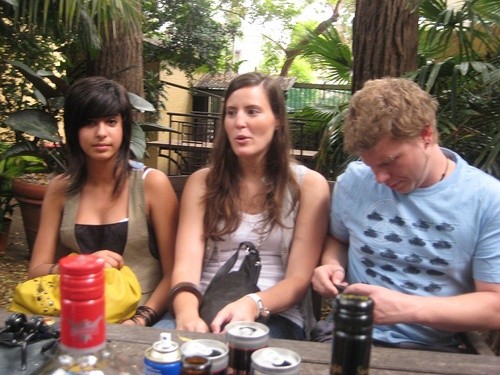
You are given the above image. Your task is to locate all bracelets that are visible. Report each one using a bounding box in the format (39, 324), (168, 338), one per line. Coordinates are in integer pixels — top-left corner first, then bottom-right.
(48, 263), (58, 275)
(169, 282), (203, 319)
(247, 293), (271, 321)
(131, 306), (158, 327)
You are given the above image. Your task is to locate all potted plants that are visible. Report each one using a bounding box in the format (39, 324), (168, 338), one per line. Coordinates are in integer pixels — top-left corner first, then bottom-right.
(0, 141), (47, 257)
(2, 56), (184, 259)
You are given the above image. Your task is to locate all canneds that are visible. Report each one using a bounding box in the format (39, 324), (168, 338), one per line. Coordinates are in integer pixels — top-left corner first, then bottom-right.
(222, 321), (270, 375)
(179, 338), (229, 375)
(250, 347), (302, 375)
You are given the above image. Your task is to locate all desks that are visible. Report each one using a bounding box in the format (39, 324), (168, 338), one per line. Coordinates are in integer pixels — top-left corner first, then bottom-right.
(0, 308), (500, 375)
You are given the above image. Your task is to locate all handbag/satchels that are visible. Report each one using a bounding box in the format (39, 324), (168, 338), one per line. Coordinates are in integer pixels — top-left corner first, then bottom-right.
(198, 242), (262, 334)
(11, 266), (141, 323)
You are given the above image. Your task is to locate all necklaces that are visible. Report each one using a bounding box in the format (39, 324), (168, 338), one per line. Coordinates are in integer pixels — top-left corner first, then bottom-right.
(438, 158), (450, 182)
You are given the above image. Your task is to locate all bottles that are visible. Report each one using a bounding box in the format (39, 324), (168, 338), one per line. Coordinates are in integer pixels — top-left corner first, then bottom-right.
(30, 253), (146, 374)
(330, 293), (375, 375)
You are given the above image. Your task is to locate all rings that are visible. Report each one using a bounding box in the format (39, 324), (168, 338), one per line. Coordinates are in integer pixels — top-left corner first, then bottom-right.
(332, 269), (345, 274)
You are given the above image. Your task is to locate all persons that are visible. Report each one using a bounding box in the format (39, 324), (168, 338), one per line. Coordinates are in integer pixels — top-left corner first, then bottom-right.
(150, 71), (331, 343)
(311, 76), (500, 352)
(26, 77), (180, 333)
